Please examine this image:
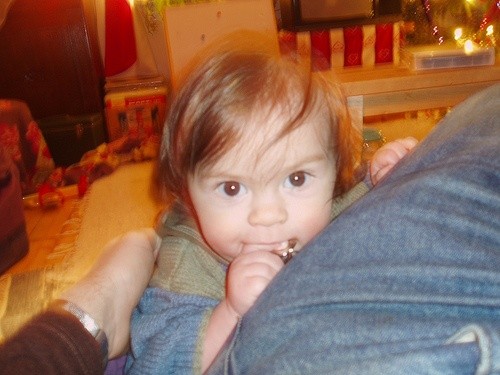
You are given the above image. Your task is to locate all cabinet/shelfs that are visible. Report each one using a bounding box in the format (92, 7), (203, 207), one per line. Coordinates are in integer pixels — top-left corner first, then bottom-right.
(313, 55), (500, 166)
(0, 0), (104, 113)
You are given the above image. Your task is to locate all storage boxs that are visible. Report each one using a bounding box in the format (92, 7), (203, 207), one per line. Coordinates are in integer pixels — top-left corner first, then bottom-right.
(38, 115), (105, 166)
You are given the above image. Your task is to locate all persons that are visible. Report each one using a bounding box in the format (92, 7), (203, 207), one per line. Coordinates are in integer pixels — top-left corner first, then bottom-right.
(1, 81), (500, 375)
(122, 45), (418, 375)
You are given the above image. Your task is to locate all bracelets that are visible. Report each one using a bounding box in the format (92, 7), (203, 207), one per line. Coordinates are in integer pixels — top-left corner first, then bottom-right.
(46, 300), (109, 373)
